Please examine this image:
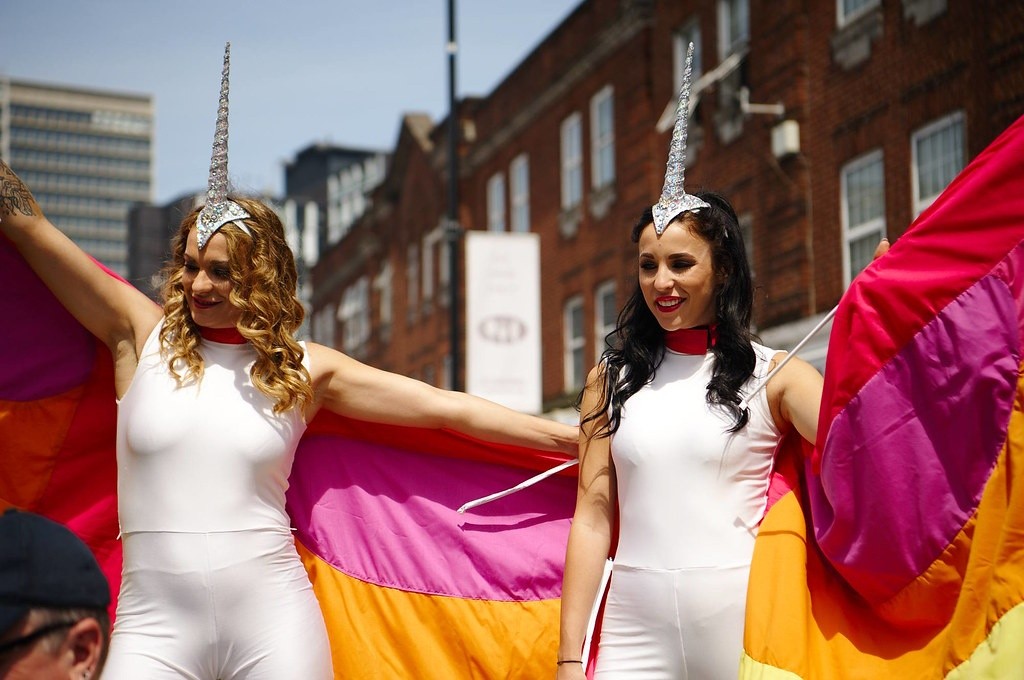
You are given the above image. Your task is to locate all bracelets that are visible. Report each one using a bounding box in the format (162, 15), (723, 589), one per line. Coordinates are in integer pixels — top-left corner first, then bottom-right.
(556, 660), (583, 665)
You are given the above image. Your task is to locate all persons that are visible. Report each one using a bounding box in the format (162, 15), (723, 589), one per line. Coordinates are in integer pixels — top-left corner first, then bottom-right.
(1, 509), (113, 679)
(559, 192), (826, 680)
(0, 159), (579, 679)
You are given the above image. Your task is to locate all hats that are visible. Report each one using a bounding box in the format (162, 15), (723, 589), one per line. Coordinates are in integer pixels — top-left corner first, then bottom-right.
(0, 508), (111, 610)
